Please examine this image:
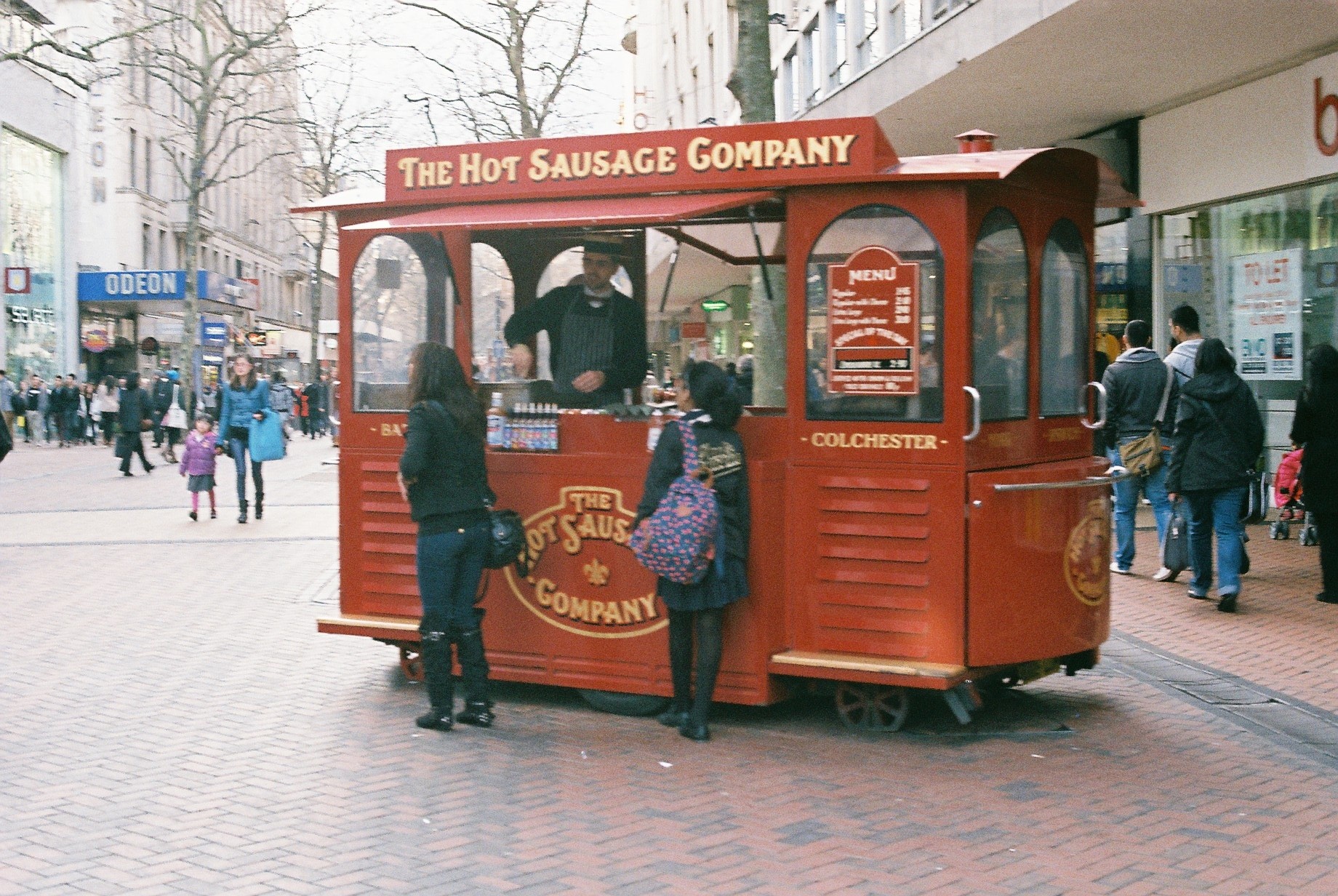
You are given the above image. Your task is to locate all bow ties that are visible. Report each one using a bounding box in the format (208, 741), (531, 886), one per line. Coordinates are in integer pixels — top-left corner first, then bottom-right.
(588, 293), (610, 305)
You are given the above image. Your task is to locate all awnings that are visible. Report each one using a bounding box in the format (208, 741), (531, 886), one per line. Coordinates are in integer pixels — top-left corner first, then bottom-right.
(340, 189), (781, 346)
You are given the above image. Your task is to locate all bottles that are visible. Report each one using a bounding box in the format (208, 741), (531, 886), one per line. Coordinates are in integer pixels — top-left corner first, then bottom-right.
(645, 409), (666, 459)
(485, 392), (558, 455)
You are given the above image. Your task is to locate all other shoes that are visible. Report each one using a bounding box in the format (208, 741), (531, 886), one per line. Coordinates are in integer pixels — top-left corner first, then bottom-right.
(146, 464), (156, 472)
(1239, 537), (1250, 575)
(1219, 594), (1235, 612)
(25, 438), (113, 448)
(456, 704), (491, 727)
(125, 472), (133, 476)
(1316, 589), (1338, 603)
(238, 512), (247, 523)
(211, 510), (216, 518)
(680, 721), (710, 742)
(256, 505), (262, 520)
(416, 707), (451, 731)
(1188, 587), (1205, 599)
(655, 705), (688, 725)
(190, 512), (197, 520)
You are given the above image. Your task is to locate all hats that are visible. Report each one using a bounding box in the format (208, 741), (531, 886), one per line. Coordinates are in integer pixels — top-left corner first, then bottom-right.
(569, 234), (636, 264)
(166, 370), (178, 381)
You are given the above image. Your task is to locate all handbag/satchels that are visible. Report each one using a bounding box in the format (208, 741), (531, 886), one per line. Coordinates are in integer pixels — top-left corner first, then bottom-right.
(161, 403), (189, 431)
(1165, 498), (1188, 571)
(485, 510), (523, 567)
(115, 436), (125, 459)
(247, 409), (283, 462)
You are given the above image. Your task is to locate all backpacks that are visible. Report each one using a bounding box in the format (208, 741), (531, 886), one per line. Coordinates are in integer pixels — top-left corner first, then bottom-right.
(630, 419), (724, 585)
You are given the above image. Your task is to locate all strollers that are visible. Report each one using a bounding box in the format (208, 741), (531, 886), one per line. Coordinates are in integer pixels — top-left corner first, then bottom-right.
(1270, 448), (1320, 547)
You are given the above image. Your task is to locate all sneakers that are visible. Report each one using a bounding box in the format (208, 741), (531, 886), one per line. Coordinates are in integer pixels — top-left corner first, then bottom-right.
(1153, 566), (1181, 581)
(1109, 562), (1128, 574)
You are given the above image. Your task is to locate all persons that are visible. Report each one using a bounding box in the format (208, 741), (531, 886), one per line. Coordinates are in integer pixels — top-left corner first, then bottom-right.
(1094, 320), (1178, 581)
(807, 350), (827, 401)
(1164, 305), (1250, 574)
(1094, 350), (1109, 387)
(919, 340), (939, 388)
(724, 353), (754, 405)
(647, 366), (676, 388)
(1166, 339), (1264, 613)
(0, 365), (126, 447)
(257, 371), (339, 447)
(214, 353), (272, 522)
(140, 366), (217, 463)
(633, 360), (751, 740)
(118, 372), (155, 477)
(179, 414), (222, 521)
(504, 240), (649, 406)
(396, 344), (497, 728)
(976, 319), (1024, 384)
(1288, 346), (1338, 605)
